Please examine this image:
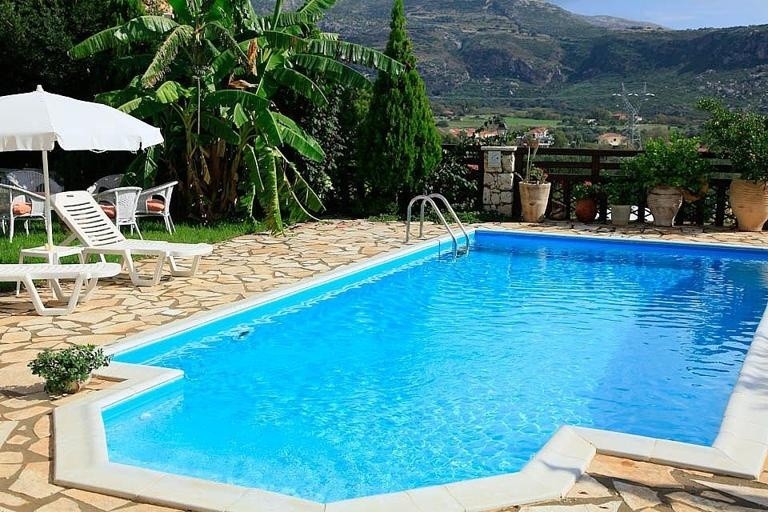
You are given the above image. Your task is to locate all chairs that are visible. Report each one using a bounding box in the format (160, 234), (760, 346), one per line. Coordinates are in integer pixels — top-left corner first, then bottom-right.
(0, 262), (120, 315)
(85, 172), (125, 193)
(95, 186), (144, 241)
(51, 186), (208, 286)
(131, 180), (179, 235)
(0, 182), (48, 238)
(7, 169), (64, 195)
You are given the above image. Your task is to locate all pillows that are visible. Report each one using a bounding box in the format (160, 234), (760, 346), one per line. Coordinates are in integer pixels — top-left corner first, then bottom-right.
(14, 203), (32, 215)
(99, 205), (116, 217)
(147, 199), (165, 212)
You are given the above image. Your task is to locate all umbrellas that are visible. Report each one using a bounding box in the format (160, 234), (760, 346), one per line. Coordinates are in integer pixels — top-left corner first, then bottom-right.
(0, 84), (165, 267)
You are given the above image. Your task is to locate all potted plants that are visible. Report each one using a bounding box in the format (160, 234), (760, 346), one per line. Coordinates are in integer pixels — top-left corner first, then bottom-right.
(696, 90), (767, 231)
(628, 129), (712, 228)
(573, 183), (599, 220)
(28, 340), (111, 395)
(604, 183), (635, 226)
(512, 130), (550, 221)
